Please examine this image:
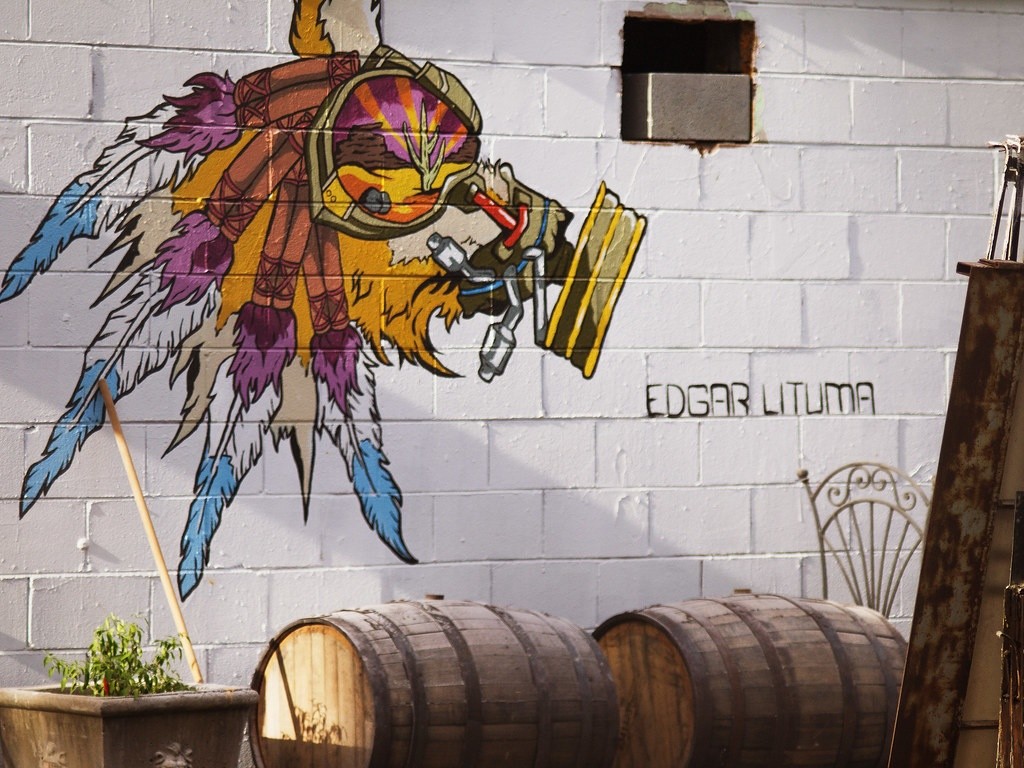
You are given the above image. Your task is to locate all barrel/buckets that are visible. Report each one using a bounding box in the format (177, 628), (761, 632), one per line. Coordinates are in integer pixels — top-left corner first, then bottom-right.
(588, 592), (906, 768)
(251, 596), (620, 768)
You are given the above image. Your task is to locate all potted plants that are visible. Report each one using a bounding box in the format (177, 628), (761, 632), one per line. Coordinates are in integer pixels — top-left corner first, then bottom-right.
(0, 611), (259, 768)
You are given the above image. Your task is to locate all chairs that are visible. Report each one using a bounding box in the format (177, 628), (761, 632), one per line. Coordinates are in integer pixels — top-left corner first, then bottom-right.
(797, 459), (937, 619)
